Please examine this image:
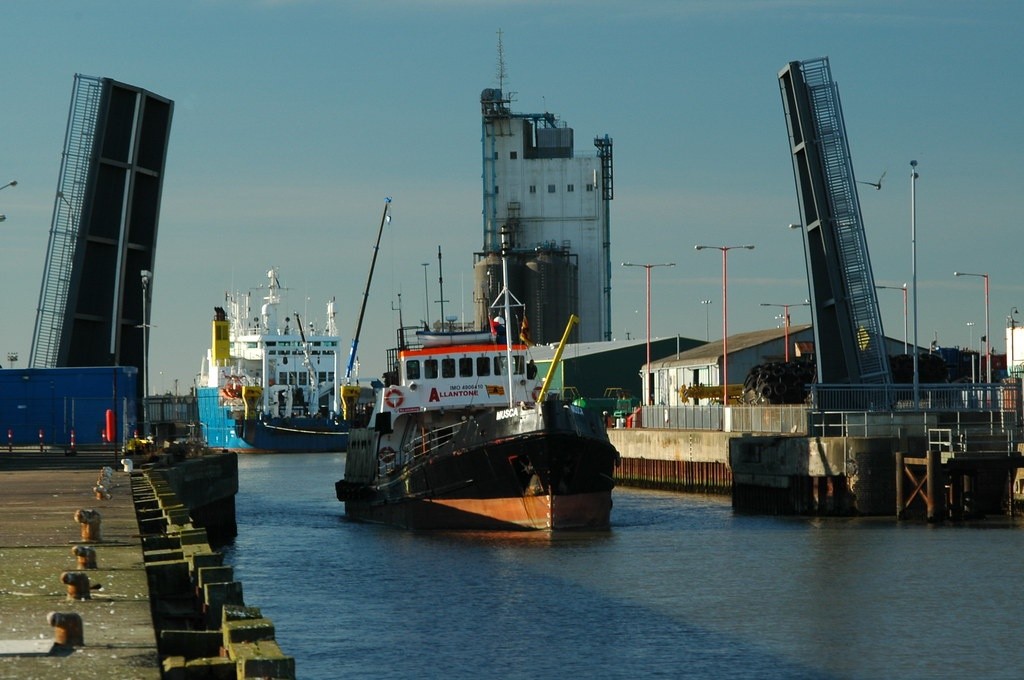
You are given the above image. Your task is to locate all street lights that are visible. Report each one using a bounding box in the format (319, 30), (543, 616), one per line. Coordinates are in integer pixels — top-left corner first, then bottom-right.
(954, 271), (991, 383)
(621, 262), (676, 408)
(759, 303), (811, 362)
(694, 244), (754, 406)
(875, 284), (908, 354)
(1009, 306), (1019, 379)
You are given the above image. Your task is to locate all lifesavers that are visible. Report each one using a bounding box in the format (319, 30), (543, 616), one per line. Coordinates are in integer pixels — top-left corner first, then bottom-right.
(386, 390), (404, 407)
(532, 386), (543, 402)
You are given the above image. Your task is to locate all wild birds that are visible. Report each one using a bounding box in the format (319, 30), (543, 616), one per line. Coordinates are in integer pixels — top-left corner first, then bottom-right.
(855, 170), (888, 190)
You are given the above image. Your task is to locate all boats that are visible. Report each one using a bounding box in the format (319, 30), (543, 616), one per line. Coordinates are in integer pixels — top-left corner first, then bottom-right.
(335, 227), (621, 539)
(234, 376), (383, 452)
